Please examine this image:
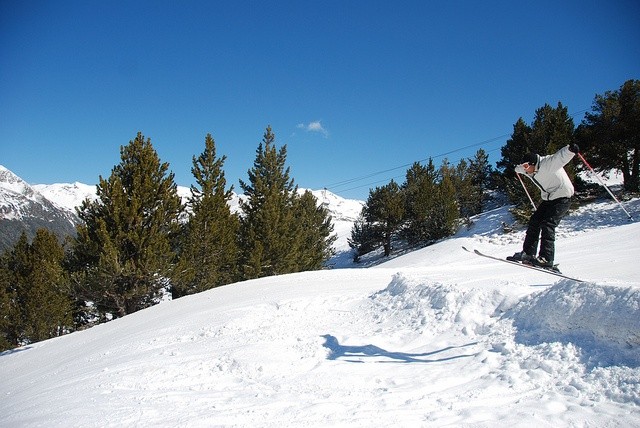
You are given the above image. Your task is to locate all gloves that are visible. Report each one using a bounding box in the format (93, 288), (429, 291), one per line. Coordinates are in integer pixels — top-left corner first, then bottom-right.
(569, 142), (580, 155)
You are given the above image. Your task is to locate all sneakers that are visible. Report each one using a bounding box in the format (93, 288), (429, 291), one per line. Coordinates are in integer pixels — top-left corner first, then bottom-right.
(507, 250), (535, 262)
(522, 256), (553, 266)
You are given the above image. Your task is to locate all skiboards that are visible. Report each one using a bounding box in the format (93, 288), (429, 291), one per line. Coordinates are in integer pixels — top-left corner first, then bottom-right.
(462, 246), (583, 282)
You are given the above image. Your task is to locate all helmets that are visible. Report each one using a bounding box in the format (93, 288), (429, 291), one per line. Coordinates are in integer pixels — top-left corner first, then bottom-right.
(520, 154), (537, 165)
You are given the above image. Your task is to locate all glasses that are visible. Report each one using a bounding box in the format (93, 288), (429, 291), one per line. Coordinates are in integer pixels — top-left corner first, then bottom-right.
(520, 162), (532, 169)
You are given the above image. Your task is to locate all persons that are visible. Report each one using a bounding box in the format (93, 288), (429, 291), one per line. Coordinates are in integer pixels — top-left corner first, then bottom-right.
(506, 144), (582, 268)
